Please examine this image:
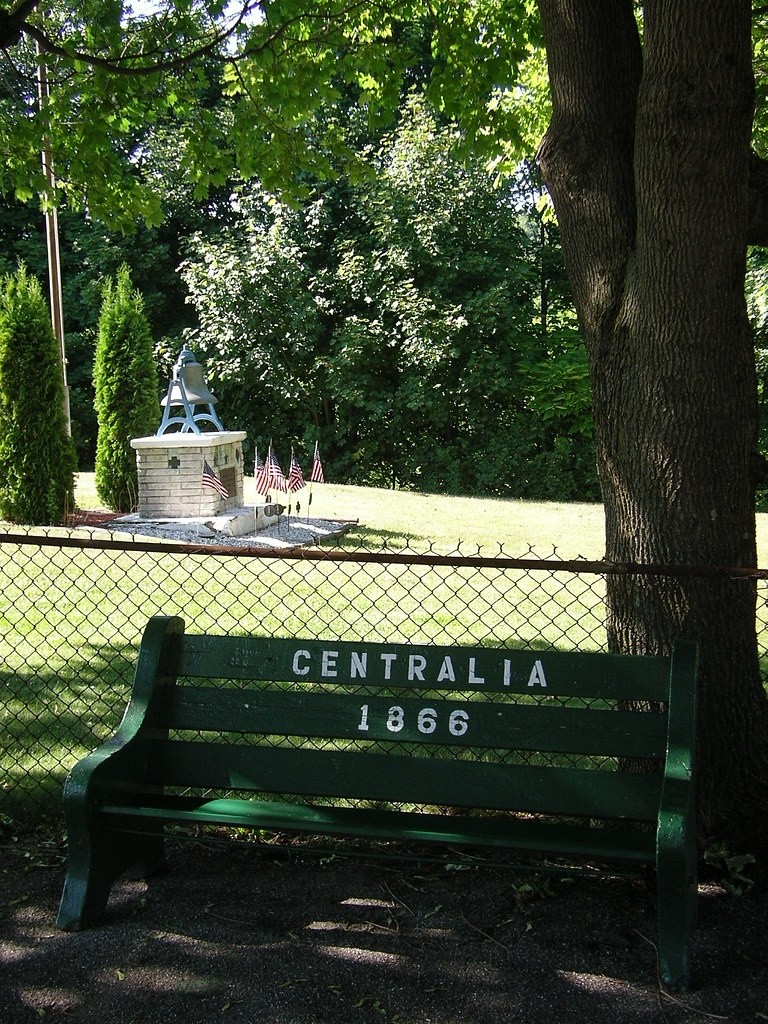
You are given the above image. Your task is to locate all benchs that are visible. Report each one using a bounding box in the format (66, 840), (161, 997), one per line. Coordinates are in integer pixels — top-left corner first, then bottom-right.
(51, 617), (698, 986)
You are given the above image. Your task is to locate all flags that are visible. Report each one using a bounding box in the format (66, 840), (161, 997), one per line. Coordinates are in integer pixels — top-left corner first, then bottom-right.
(265, 453), (287, 494)
(311, 442), (324, 483)
(288, 451), (307, 494)
(254, 452), (270, 496)
(202, 460), (229, 501)
